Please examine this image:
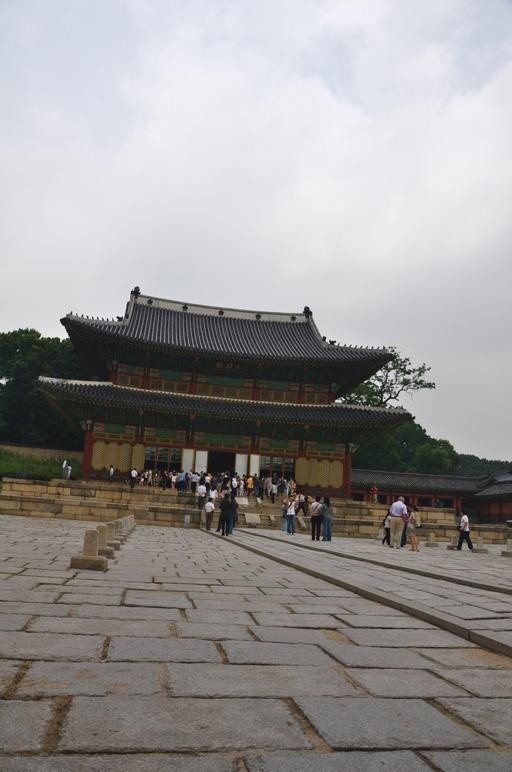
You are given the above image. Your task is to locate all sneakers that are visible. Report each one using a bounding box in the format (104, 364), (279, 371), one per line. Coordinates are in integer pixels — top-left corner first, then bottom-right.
(286, 530), (294, 534)
(312, 536), (332, 542)
(381, 538), (420, 553)
(205, 526), (231, 538)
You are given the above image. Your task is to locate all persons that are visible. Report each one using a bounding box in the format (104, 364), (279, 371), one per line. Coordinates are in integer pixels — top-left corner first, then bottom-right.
(318, 496), (333, 541)
(453, 510), (473, 551)
(382, 503), (420, 552)
(62, 459), (72, 480)
(389, 495), (408, 549)
(311, 495), (322, 541)
(88, 465), (315, 536)
(371, 485), (378, 504)
